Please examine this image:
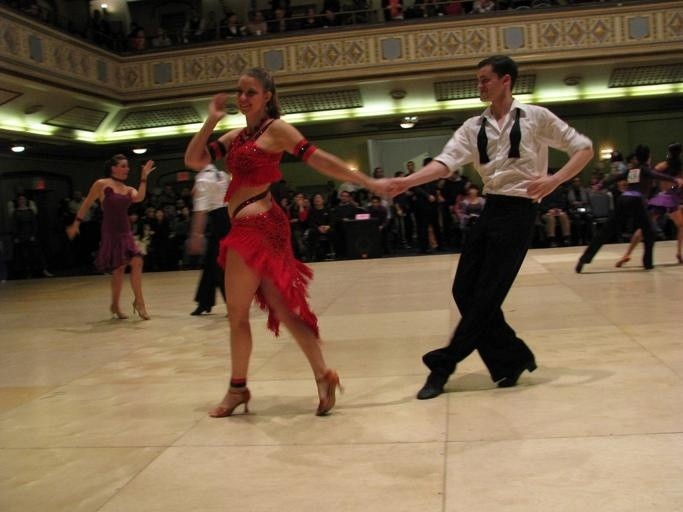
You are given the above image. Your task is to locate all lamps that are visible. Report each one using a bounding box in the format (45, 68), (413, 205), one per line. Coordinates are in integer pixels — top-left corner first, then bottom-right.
(100, 2), (111, 17)
(7, 142), (28, 154)
(398, 115), (418, 129)
(132, 147), (147, 155)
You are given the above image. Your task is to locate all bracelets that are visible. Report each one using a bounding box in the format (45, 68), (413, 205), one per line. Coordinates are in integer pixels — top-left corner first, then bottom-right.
(139, 178), (146, 183)
(74, 215), (82, 222)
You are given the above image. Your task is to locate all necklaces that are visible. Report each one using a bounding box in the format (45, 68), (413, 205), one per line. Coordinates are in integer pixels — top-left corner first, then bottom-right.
(238, 115), (269, 145)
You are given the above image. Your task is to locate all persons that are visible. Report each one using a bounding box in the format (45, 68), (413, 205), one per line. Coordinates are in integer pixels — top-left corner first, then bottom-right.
(182, 149), (233, 314)
(0, 141), (682, 274)
(382, 54), (596, 399)
(63, 151), (159, 321)
(0, 1), (600, 55)
(184, 67), (402, 420)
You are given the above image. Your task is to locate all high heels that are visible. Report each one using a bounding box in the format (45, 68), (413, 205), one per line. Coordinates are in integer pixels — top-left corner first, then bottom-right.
(315, 369), (343, 416)
(132, 299), (150, 320)
(209, 388), (250, 418)
(497, 357), (537, 388)
(191, 303), (211, 315)
(418, 375), (445, 399)
(111, 304), (129, 319)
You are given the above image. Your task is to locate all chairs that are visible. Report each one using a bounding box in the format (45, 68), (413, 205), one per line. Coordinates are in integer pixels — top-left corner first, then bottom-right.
(585, 189), (611, 225)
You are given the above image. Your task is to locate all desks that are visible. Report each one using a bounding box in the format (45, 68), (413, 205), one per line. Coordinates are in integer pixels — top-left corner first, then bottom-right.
(338, 214), (382, 262)
